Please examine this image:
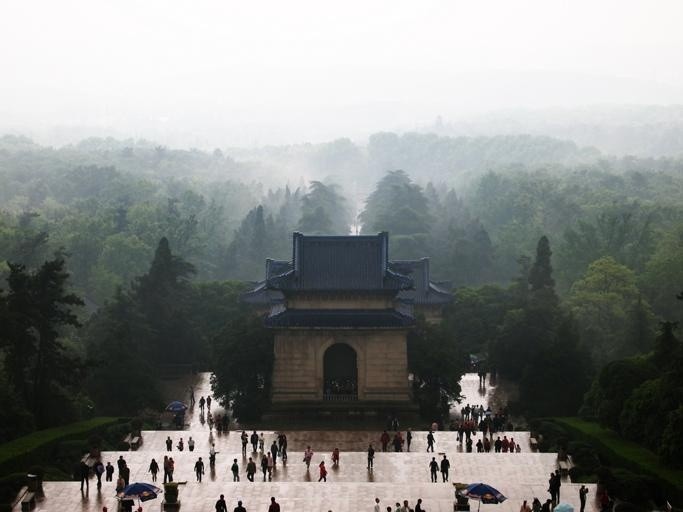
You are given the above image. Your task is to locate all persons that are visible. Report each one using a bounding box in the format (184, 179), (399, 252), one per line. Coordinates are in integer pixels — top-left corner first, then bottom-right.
(374, 497), (426, 512)
(380, 413), (444, 453)
(188, 385), (230, 431)
(324, 375), (358, 400)
(153, 411), (185, 430)
(454, 494), (470, 511)
(231, 430), (287, 482)
(471, 360), (489, 383)
(268, 497), (280, 512)
(328, 510), (332, 512)
(429, 454), (451, 483)
(233, 501), (246, 512)
(78, 436), (216, 512)
(520, 469), (589, 512)
(448, 402), (521, 452)
(303, 443), (376, 483)
(215, 494), (227, 512)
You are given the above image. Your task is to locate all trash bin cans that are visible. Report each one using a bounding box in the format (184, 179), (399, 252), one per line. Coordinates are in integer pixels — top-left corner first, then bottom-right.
(27, 474), (38, 491)
